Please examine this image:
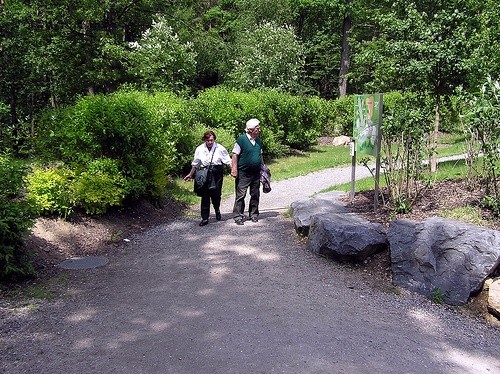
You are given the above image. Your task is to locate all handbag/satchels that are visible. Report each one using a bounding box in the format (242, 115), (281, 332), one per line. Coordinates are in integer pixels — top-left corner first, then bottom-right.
(193, 168), (216, 193)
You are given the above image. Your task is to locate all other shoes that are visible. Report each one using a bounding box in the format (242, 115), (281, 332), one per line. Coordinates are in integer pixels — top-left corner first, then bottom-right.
(250, 216), (258, 222)
(236, 219), (244, 225)
(215, 210), (221, 220)
(199, 220), (209, 226)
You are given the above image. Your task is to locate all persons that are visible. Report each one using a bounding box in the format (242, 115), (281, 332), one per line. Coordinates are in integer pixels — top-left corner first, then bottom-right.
(183, 130), (233, 226)
(230, 118), (266, 225)
